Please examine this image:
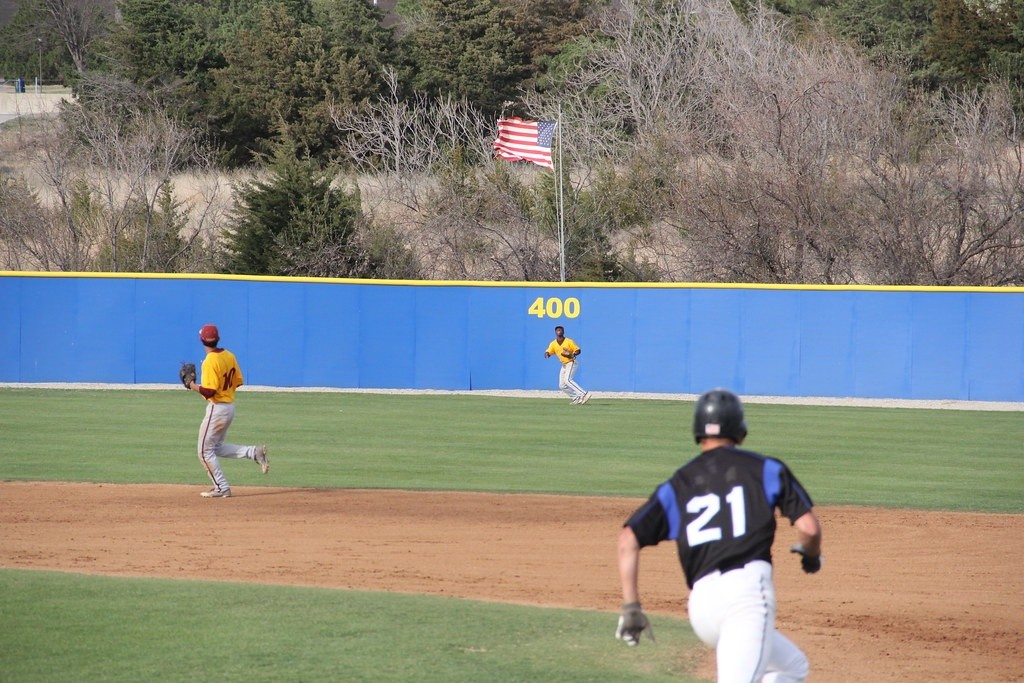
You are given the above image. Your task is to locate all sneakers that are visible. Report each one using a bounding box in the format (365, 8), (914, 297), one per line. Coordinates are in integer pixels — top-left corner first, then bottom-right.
(199, 487), (232, 498)
(256, 444), (269, 476)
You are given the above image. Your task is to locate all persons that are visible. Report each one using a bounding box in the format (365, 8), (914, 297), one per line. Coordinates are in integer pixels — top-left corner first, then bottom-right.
(180, 325), (270, 497)
(615, 390), (822, 683)
(545, 326), (592, 405)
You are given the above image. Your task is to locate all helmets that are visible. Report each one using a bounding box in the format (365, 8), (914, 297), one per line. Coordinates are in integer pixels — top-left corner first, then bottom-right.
(692, 389), (748, 445)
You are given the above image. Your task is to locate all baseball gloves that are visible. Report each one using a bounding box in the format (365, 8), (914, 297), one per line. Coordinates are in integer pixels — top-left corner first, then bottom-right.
(561, 347), (576, 363)
(178, 360), (196, 392)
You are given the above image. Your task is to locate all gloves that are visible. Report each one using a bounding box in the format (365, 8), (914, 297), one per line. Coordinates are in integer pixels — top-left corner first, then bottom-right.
(788, 545), (821, 574)
(614, 602), (655, 651)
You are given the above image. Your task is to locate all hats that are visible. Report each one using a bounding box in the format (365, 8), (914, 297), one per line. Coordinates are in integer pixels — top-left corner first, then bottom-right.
(199, 325), (220, 342)
(554, 325), (564, 333)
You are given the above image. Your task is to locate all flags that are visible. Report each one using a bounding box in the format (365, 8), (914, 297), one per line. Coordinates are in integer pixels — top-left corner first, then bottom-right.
(494, 117), (557, 170)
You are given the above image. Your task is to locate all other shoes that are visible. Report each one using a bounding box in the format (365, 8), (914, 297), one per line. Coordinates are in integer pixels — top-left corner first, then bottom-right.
(569, 397), (583, 406)
(581, 391), (592, 405)
